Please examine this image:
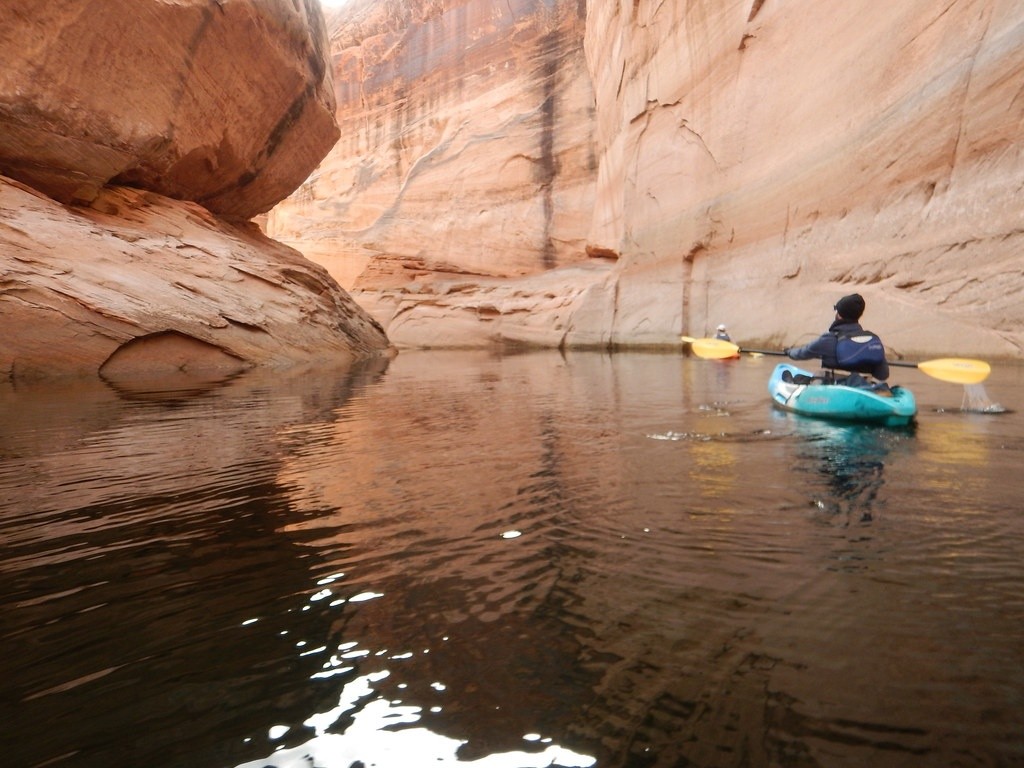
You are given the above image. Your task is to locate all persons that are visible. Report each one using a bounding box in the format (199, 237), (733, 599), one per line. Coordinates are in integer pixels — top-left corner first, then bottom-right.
(714, 324), (730, 342)
(783, 293), (890, 391)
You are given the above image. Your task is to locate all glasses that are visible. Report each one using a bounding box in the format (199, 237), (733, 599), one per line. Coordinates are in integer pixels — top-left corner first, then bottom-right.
(834, 305), (839, 311)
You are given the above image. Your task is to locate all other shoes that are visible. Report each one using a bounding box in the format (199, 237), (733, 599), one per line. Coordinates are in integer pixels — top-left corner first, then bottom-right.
(783, 348), (790, 355)
(782, 370), (793, 384)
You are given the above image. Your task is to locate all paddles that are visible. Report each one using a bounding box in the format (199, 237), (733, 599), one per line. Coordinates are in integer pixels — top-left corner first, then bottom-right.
(680, 336), (763, 359)
(693, 339), (990, 384)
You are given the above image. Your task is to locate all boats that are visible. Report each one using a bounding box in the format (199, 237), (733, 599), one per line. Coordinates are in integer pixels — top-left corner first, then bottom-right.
(715, 353), (741, 359)
(767, 362), (917, 428)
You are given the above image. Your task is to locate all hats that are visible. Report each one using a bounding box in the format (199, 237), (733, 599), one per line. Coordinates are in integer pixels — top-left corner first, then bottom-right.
(836, 293), (865, 321)
(716, 324), (725, 331)
